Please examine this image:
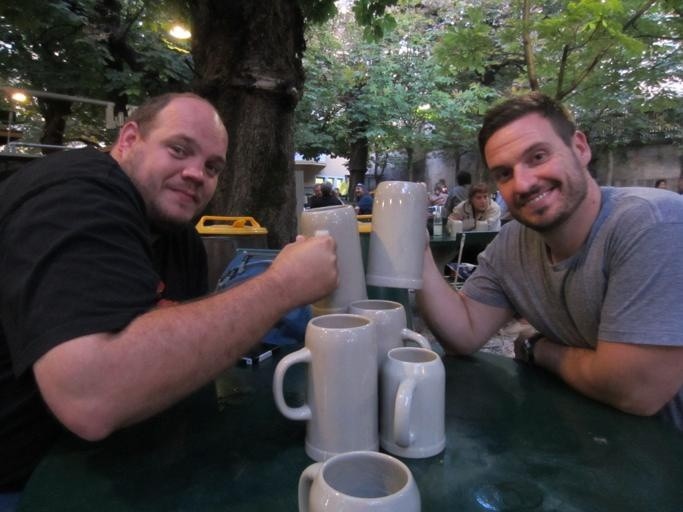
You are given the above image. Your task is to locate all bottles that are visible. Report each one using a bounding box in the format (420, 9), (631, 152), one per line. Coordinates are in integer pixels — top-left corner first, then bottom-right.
(432, 205), (443, 238)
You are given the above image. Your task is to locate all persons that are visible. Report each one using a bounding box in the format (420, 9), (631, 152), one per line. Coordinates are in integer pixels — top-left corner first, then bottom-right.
(421, 92), (683, 425)
(309, 179), (372, 217)
(0, 92), (340, 512)
(430, 171), (511, 230)
(655, 178), (668, 189)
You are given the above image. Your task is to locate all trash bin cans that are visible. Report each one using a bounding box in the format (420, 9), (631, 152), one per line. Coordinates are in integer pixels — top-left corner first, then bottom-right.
(354, 215), (372, 273)
(427, 206), (435, 237)
(194, 215), (270, 289)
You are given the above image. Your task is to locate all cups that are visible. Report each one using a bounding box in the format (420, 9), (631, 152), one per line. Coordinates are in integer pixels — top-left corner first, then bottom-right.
(365, 179), (428, 290)
(272, 314), (381, 463)
(449, 220), (463, 241)
(476, 221), (487, 232)
(347, 299), (431, 376)
(295, 450), (421, 512)
(297, 201), (366, 315)
(378, 345), (448, 460)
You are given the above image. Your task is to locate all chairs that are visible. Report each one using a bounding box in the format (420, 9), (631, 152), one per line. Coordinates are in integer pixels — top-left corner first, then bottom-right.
(445, 228), (498, 292)
(215, 244), (313, 368)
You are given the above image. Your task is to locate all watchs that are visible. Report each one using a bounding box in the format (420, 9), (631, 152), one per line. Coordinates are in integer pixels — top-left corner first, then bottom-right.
(516, 332), (546, 367)
